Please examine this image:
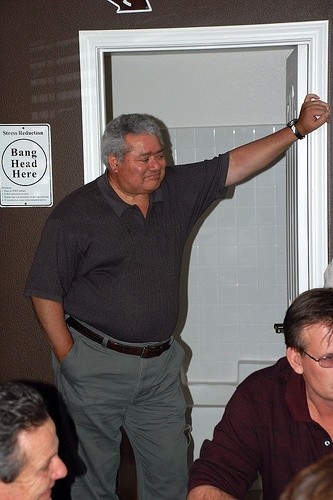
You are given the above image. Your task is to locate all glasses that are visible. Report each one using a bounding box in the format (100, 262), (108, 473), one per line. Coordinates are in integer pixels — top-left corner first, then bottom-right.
(301, 349), (333, 368)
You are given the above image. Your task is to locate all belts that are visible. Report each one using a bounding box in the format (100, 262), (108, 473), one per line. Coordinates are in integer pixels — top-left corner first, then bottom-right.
(67, 317), (173, 359)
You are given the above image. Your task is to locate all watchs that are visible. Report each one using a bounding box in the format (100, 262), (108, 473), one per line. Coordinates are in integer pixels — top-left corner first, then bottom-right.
(287, 119), (305, 140)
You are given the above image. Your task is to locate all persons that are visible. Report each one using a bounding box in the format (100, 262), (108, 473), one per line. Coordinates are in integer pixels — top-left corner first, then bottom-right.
(23, 94), (333, 500)
(186, 288), (333, 500)
(0, 381), (68, 500)
